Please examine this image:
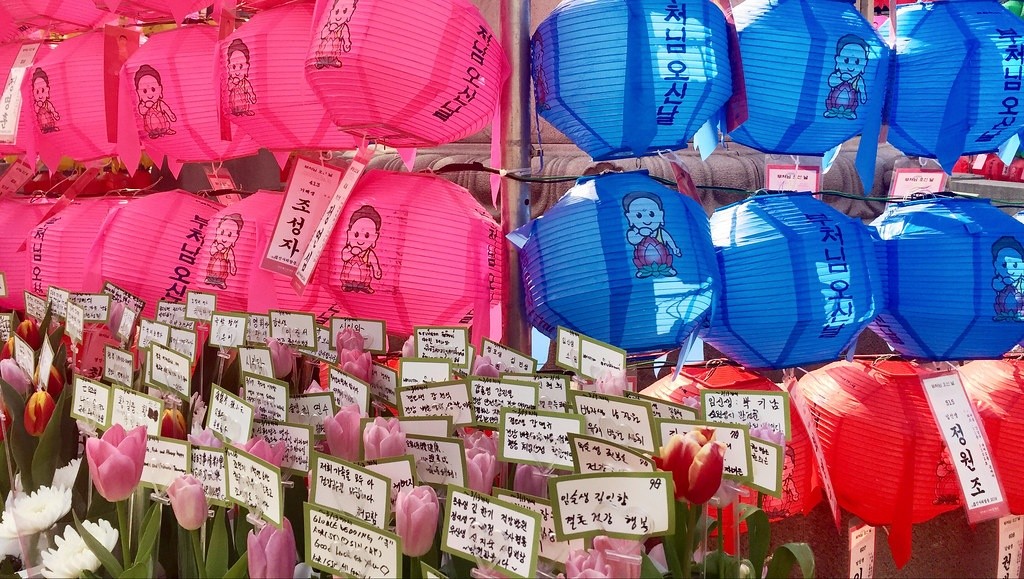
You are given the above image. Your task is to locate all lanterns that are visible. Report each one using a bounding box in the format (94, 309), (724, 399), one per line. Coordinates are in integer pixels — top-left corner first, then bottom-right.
(0, 0), (1024, 563)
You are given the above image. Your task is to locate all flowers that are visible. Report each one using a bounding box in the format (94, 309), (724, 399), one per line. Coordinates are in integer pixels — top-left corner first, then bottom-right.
(0, 303), (816, 579)
